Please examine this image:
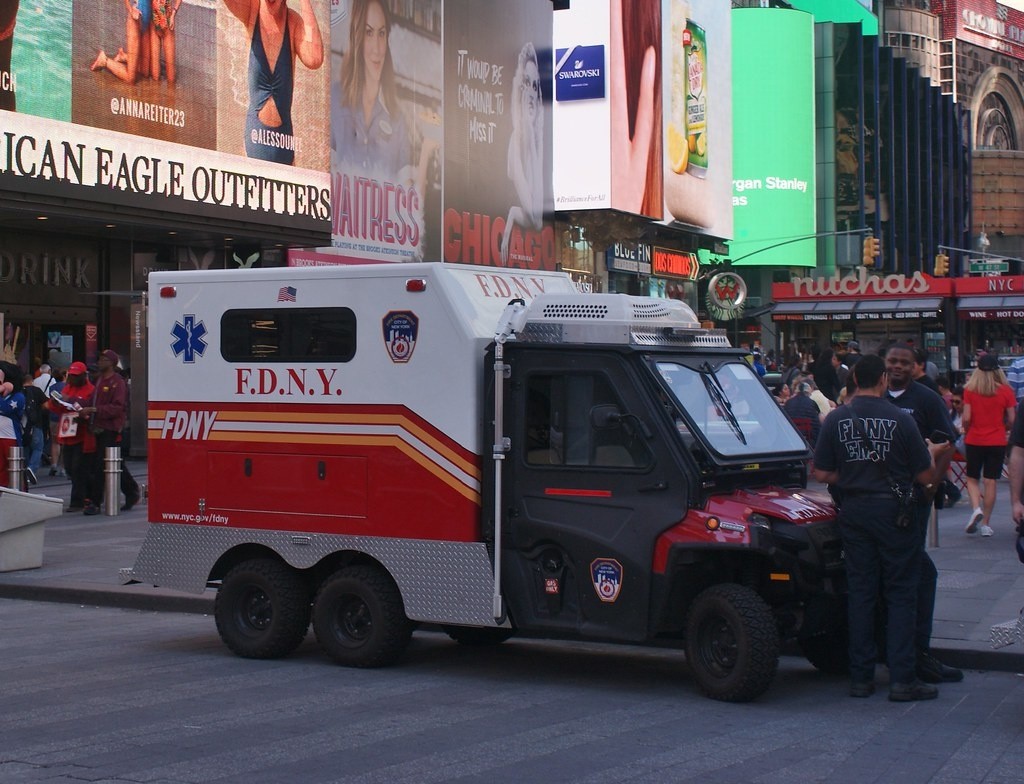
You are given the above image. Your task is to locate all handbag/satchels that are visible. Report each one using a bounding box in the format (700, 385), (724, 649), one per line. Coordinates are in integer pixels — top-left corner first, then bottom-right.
(29, 405), (49, 427)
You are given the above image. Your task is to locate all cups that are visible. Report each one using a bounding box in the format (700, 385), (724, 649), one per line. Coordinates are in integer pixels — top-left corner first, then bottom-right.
(929, 430), (950, 444)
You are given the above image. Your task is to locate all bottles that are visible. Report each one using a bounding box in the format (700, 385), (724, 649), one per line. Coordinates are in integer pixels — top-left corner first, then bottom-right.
(969, 354), (979, 368)
(664, 1), (719, 231)
(989, 324), (1024, 354)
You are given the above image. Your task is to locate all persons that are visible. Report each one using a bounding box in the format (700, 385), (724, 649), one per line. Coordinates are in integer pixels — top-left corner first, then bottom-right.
(33, 364), (57, 399)
(22, 374), (48, 485)
(911, 349), (964, 452)
(331, 0), (438, 201)
(48, 361), (96, 512)
(961, 354), (1018, 535)
(1005, 396), (1024, 564)
(814, 354), (963, 703)
(223, 0), (324, 166)
(49, 367), (68, 476)
(91, 0), (184, 84)
(80, 350), (141, 514)
(881, 342), (957, 545)
(1007, 356), (1024, 403)
(750, 342), (862, 443)
(501, 42), (544, 266)
(0, 361), (26, 489)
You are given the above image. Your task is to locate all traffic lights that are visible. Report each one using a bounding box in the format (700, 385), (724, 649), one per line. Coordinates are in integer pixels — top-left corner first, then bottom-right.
(863, 237), (881, 267)
(933, 254), (950, 276)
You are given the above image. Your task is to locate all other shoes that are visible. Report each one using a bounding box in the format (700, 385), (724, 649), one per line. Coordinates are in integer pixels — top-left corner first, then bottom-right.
(851, 680), (874, 697)
(83, 504), (101, 515)
(917, 654), (965, 681)
(26, 467), (38, 485)
(121, 496), (142, 510)
(49, 467), (57, 476)
(946, 492), (962, 508)
(60, 469), (65, 477)
(66, 506), (82, 512)
(889, 680), (939, 701)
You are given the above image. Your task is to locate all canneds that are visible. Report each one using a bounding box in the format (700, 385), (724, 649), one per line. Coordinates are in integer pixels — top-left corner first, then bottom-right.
(682, 18), (709, 179)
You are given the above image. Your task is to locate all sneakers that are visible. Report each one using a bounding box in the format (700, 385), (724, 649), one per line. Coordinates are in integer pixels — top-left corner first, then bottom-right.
(981, 525), (993, 536)
(965, 507), (984, 533)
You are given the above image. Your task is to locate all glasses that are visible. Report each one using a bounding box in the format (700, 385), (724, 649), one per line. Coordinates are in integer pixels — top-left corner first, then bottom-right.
(950, 399), (961, 405)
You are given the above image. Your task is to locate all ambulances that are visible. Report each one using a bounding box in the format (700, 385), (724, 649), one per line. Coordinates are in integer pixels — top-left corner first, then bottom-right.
(116, 260), (848, 703)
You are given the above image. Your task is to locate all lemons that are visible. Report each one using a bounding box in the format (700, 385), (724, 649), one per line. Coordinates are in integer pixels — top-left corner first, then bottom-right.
(668, 124), (688, 174)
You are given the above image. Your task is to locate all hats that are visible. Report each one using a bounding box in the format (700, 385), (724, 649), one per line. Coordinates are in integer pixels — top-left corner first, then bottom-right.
(101, 349), (119, 366)
(67, 362), (87, 375)
(849, 341), (861, 352)
(979, 355), (1000, 371)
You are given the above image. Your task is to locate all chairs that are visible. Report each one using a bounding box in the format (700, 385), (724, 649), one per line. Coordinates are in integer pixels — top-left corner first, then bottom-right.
(530, 448), (563, 467)
(597, 444), (637, 468)
(949, 454), (985, 501)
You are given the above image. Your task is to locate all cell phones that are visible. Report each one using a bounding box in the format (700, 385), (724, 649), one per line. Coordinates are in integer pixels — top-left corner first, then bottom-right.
(931, 430), (951, 444)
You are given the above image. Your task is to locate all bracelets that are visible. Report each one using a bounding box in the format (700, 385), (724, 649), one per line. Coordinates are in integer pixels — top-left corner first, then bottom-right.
(174, 8), (177, 13)
